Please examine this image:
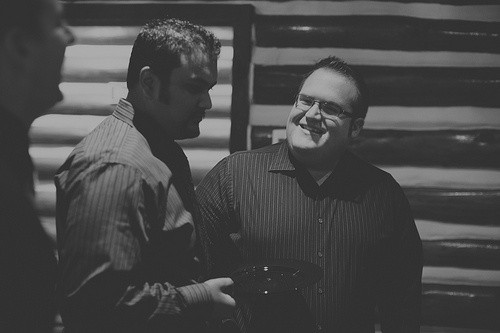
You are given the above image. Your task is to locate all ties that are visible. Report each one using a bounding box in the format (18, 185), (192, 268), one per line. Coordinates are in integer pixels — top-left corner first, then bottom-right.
(161, 133), (217, 281)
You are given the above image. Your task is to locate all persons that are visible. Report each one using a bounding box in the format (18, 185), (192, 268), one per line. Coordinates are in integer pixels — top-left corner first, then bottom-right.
(0, 0), (76, 333)
(52, 19), (238, 333)
(194, 56), (425, 333)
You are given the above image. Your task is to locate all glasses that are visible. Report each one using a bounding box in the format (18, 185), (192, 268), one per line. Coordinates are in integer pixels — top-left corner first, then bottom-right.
(295, 93), (360, 121)
